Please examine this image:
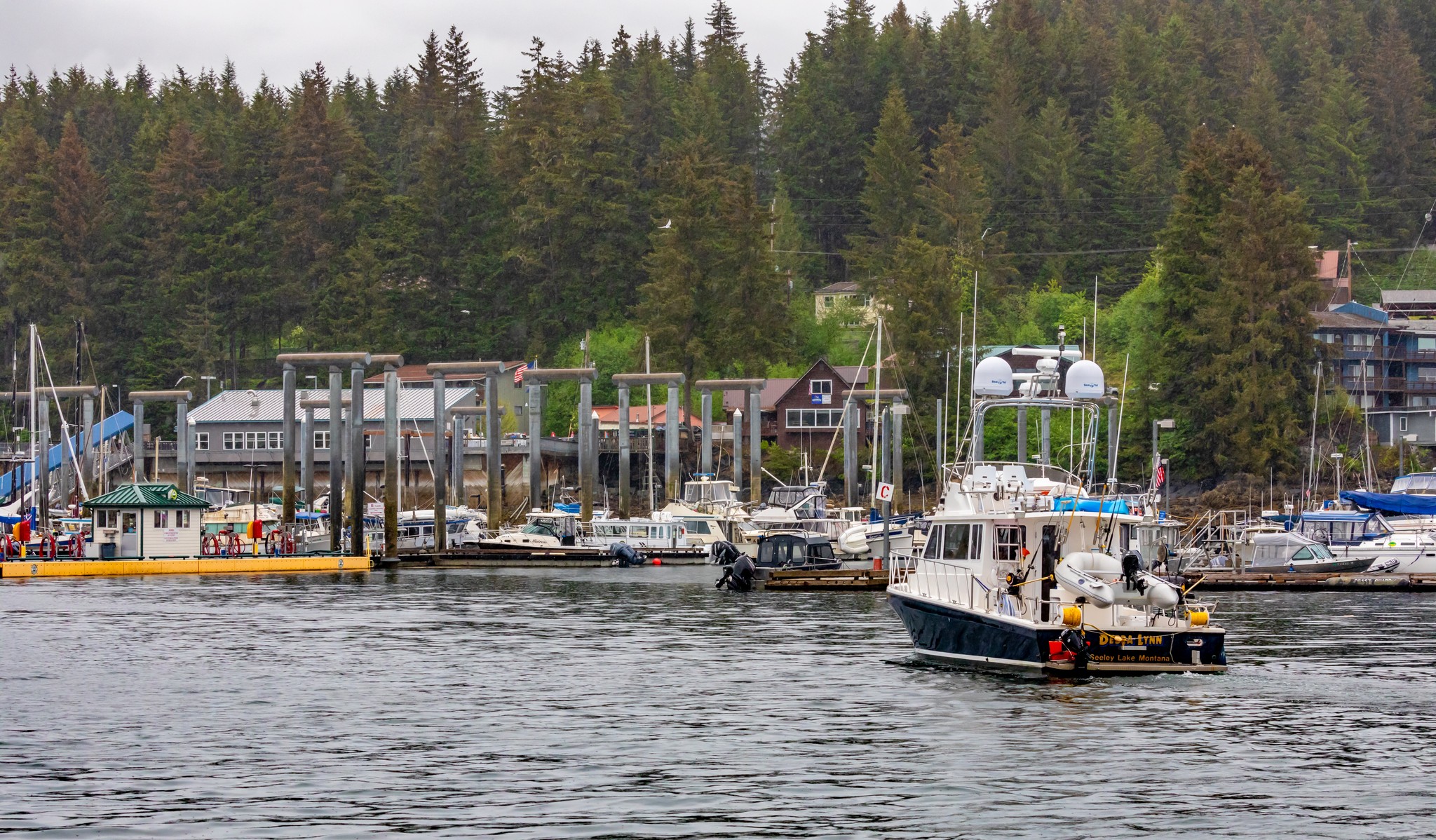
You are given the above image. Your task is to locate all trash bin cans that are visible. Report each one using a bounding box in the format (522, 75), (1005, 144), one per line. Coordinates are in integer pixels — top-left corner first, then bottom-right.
(873, 555), (882, 571)
(101, 542), (115, 559)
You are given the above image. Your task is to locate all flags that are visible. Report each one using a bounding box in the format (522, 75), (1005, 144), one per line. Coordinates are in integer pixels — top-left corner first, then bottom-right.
(514, 360), (536, 383)
(1306, 484), (1313, 496)
(1156, 456), (1164, 487)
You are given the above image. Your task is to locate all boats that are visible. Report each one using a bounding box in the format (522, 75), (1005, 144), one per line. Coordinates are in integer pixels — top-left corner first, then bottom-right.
(0, 311), (920, 592)
(883, 269), (1230, 675)
(1155, 354), (1436, 593)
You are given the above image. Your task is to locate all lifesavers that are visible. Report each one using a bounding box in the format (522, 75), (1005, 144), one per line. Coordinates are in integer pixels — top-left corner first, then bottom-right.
(1130, 508), (1142, 516)
